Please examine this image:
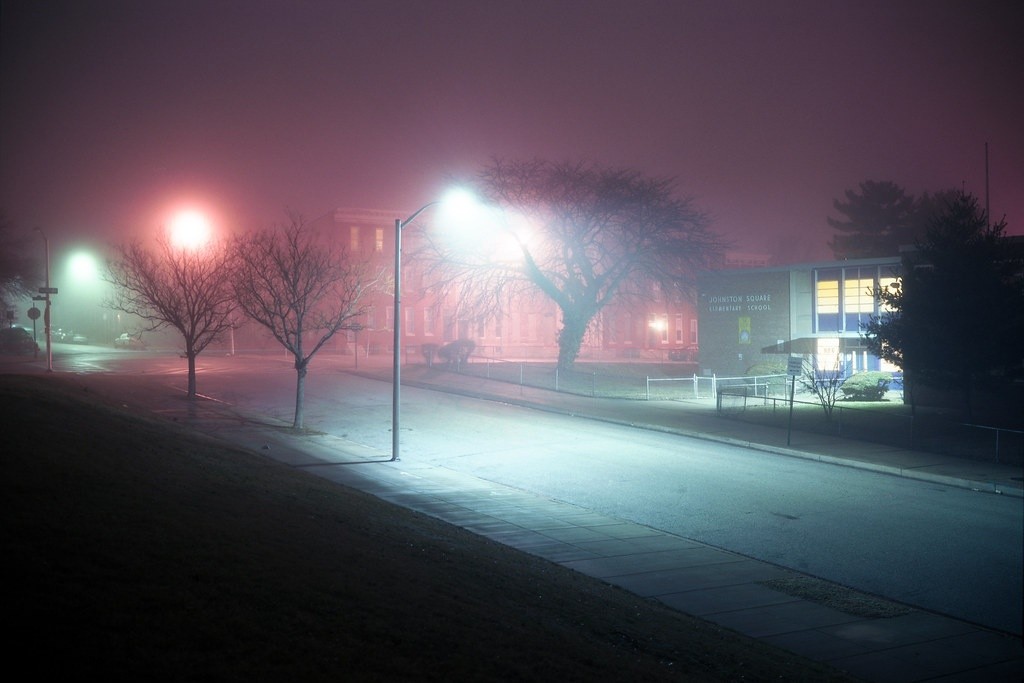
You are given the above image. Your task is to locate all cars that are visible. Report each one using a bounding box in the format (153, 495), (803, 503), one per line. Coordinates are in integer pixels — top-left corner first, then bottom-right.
(71, 333), (88, 344)
(114, 331), (147, 351)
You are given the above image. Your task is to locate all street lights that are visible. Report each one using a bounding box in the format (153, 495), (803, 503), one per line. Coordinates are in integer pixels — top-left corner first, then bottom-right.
(34, 225), (53, 371)
(389, 185), (475, 460)
